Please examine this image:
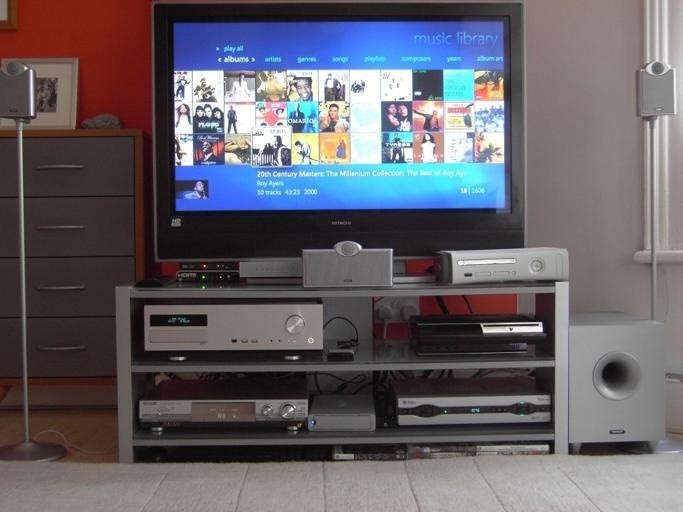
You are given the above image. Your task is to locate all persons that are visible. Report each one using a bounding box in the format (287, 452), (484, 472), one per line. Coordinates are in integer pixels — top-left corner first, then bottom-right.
(413, 107), (442, 132)
(290, 103), (317, 133)
(383, 103), (401, 132)
(174, 137), (187, 166)
(195, 142), (224, 165)
(184, 181), (207, 198)
(287, 75), (313, 101)
(258, 107), (285, 124)
(176, 72), (190, 100)
(203, 104), (212, 133)
(227, 104), (240, 133)
(386, 104), (412, 131)
(174, 104), (194, 127)
(225, 73), (255, 102)
(324, 73), (341, 101)
(193, 77), (218, 102)
(35, 79), (53, 113)
(389, 133), (409, 164)
(212, 107), (224, 133)
(260, 136), (291, 166)
(225, 138), (251, 164)
(194, 106), (206, 133)
(320, 102), (349, 134)
(294, 140), (321, 165)
(421, 133), (438, 162)
(256, 72), (286, 101)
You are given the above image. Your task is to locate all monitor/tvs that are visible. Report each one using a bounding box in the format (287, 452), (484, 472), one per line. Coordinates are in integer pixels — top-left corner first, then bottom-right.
(152, 0), (526, 284)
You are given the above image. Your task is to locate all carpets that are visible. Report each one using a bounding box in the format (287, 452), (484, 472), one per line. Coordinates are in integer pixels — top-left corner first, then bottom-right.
(0, 447), (680, 512)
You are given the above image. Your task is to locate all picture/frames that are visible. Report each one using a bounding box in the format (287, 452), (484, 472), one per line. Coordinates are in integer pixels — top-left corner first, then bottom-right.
(0, 57), (79, 130)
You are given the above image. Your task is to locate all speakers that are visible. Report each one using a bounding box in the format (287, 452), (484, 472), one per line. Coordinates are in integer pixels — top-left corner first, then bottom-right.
(566, 322), (668, 454)
(639, 62), (675, 116)
(302, 241), (393, 289)
(1, 61), (35, 118)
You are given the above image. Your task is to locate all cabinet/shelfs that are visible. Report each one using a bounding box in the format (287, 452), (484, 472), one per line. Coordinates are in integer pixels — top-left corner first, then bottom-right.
(115, 276), (569, 465)
(0, 128), (144, 412)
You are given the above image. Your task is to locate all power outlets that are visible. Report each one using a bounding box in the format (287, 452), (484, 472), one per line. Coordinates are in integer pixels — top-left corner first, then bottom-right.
(373, 297), (419, 324)
